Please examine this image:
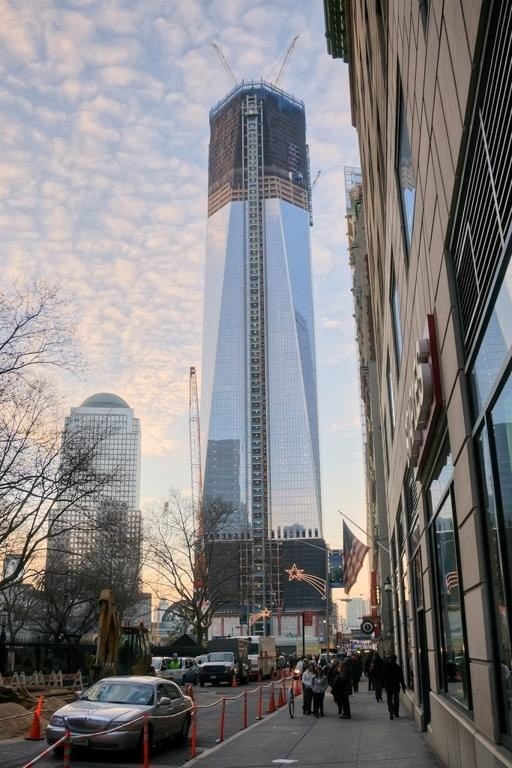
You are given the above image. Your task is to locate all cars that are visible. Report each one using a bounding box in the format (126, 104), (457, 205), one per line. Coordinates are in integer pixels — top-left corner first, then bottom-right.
(152, 653), (207, 686)
(46, 675), (194, 756)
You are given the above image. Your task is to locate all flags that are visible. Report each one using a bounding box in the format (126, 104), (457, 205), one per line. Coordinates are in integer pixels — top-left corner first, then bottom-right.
(341, 518), (371, 596)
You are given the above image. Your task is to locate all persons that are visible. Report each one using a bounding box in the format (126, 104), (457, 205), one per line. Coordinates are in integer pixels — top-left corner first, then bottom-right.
(288, 647), (408, 721)
(499, 655), (511, 711)
(169, 652), (181, 669)
(117, 639), (136, 676)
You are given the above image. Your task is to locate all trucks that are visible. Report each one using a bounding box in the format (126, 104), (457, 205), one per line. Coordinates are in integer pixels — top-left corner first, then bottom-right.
(199, 636), (277, 687)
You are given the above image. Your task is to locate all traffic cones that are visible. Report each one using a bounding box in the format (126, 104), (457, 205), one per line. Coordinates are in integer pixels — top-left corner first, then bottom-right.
(267, 691), (276, 712)
(24, 711), (44, 740)
(185, 685), (194, 701)
(276, 687), (284, 706)
(232, 673), (237, 687)
(295, 680), (299, 695)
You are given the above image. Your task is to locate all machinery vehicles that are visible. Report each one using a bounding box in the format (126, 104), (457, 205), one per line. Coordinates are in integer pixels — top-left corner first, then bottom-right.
(88, 588), (156, 683)
